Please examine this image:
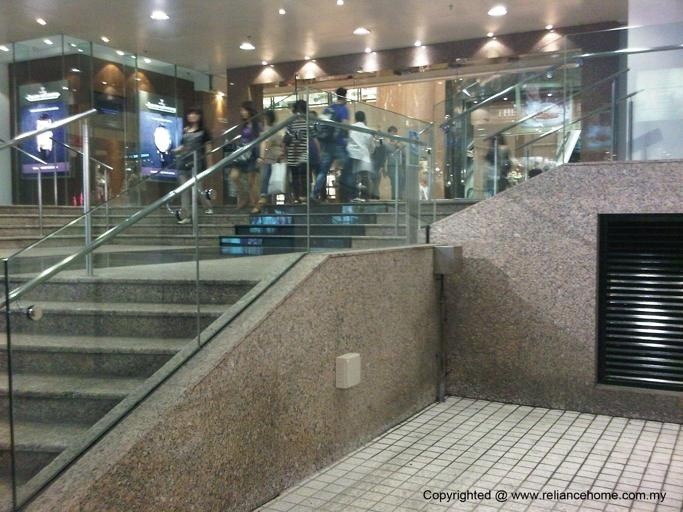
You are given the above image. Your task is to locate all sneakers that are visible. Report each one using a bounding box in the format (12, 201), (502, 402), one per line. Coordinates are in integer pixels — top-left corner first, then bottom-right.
(293, 196), (328, 204)
(350, 195), (379, 202)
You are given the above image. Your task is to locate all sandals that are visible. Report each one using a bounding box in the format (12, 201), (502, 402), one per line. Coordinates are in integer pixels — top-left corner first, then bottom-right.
(240, 197), (269, 214)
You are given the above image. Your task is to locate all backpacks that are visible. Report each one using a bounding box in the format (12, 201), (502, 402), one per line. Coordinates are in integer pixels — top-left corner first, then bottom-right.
(315, 107), (335, 141)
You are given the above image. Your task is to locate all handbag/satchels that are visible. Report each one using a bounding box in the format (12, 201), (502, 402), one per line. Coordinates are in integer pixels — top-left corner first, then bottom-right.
(267, 155), (287, 195)
(232, 145), (257, 166)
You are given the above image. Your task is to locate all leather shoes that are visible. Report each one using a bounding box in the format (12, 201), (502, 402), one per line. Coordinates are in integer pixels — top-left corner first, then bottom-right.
(177, 218), (191, 224)
(205, 209), (213, 214)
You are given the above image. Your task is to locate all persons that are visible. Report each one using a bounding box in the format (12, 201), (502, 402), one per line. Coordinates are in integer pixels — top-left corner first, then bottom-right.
(228, 87), (404, 213)
(174, 107), (215, 225)
(34, 113), (58, 162)
(481, 133), (511, 195)
(418, 156), (432, 200)
(153, 120), (172, 167)
